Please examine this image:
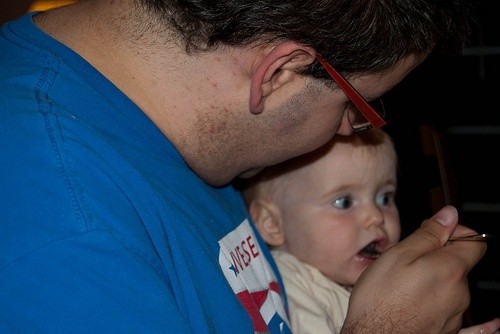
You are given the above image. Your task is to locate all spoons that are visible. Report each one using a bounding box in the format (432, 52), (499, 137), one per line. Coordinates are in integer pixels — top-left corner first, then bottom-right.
(358, 233), (489, 259)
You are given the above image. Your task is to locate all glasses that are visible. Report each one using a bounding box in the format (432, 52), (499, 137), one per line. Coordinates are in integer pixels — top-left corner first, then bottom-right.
(316, 53), (387, 132)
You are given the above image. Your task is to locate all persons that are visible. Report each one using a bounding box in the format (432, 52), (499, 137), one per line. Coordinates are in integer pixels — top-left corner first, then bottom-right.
(0, 0), (487, 334)
(241, 123), (500, 334)
